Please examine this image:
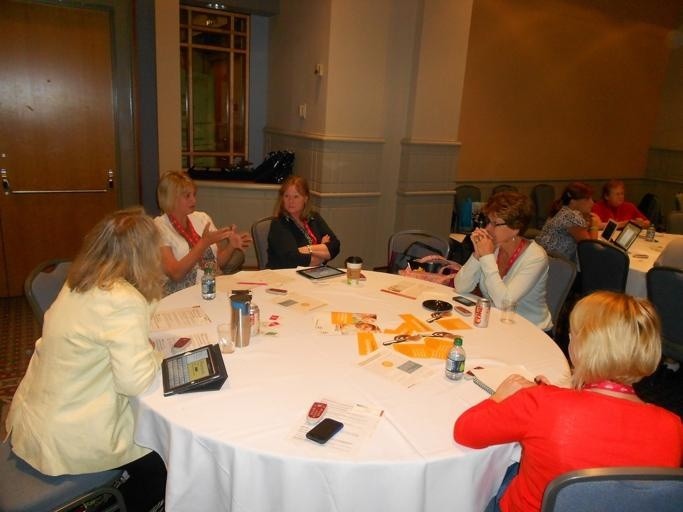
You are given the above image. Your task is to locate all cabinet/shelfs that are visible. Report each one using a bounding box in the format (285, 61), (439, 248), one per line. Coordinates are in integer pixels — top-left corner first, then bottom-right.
(187, 172), (284, 269)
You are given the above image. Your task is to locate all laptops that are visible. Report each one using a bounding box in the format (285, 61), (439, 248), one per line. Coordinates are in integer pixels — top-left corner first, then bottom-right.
(608, 220), (643, 251)
(597, 218), (618, 242)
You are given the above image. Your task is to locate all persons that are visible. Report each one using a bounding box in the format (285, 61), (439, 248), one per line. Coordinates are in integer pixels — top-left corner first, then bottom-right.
(453, 191), (554, 339)
(2, 206), (168, 511)
(151, 170), (252, 296)
(591, 178), (650, 231)
(453, 290), (683, 512)
(267, 176), (341, 271)
(534, 181), (601, 274)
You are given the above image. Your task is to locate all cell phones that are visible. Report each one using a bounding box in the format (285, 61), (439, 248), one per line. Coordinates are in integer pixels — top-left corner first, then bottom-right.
(453, 295), (476, 307)
(305, 417), (344, 445)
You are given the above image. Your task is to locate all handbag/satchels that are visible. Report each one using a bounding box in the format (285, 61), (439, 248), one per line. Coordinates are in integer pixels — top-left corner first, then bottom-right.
(396, 254), (463, 285)
(387, 241), (443, 274)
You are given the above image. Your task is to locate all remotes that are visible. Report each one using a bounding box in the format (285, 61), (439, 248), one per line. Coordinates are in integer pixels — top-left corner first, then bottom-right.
(306, 400), (328, 424)
(266, 288), (288, 295)
(360, 273), (366, 281)
(454, 306), (472, 316)
(171, 337), (192, 354)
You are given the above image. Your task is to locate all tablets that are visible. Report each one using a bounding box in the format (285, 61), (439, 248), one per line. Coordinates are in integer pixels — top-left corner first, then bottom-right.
(296, 264), (345, 281)
(162, 344), (222, 396)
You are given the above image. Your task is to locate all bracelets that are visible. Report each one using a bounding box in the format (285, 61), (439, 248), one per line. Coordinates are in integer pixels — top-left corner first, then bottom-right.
(307, 245), (312, 254)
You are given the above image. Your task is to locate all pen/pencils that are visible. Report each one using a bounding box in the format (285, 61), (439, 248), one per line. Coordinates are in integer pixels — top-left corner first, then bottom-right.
(237, 281), (267, 285)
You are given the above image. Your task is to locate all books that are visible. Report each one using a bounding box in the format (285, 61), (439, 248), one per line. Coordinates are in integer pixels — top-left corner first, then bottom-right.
(469, 364), (534, 397)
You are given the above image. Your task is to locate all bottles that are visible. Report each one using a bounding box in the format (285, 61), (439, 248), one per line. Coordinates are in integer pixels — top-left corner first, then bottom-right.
(249, 304), (260, 337)
(201, 268), (216, 300)
(230, 301), (250, 348)
(646, 223), (655, 241)
(445, 338), (466, 381)
(473, 298), (490, 328)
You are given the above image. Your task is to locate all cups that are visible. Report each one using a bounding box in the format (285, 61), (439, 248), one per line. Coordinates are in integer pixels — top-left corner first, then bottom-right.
(347, 257), (362, 286)
(500, 300), (516, 326)
(218, 323), (237, 355)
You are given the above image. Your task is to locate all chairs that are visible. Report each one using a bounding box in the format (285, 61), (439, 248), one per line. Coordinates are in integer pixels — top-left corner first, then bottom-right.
(644, 267), (683, 397)
(547, 251), (578, 338)
(577, 239), (629, 294)
(25, 257), (73, 324)
(533, 184), (554, 229)
(252, 214), (278, 270)
(494, 184), (517, 194)
(667, 193), (683, 234)
(638, 193), (661, 230)
(2, 403), (126, 512)
(540, 467), (683, 512)
(374, 230), (450, 279)
(453, 183), (482, 257)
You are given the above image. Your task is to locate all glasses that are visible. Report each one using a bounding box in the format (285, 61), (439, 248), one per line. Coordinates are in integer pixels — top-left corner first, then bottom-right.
(484, 217), (507, 227)
